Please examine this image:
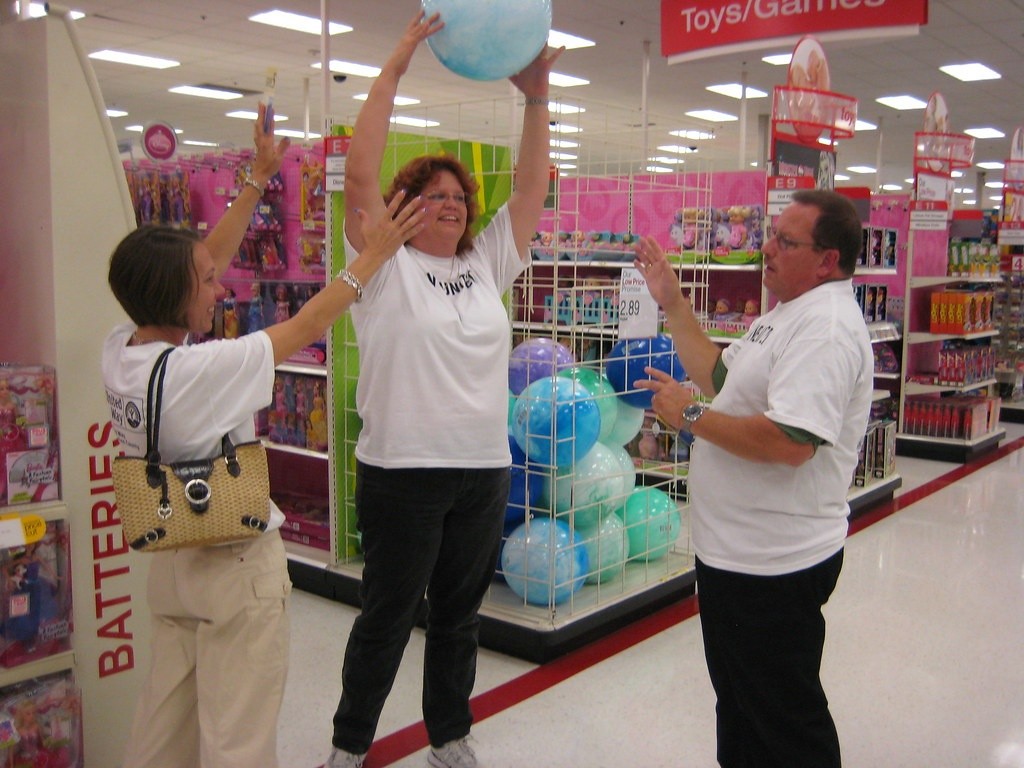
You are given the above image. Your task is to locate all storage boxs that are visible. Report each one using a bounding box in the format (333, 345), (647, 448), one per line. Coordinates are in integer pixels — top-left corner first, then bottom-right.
(854, 420), (898, 487)
(904, 396), (1000, 441)
(221, 277), (327, 367)
(857, 226), (896, 268)
(853, 281), (886, 325)
(949, 241), (999, 277)
(255, 371), (327, 456)
(272, 495), (330, 551)
(906, 346), (995, 387)
(1, 361), (85, 768)
(930, 291), (993, 334)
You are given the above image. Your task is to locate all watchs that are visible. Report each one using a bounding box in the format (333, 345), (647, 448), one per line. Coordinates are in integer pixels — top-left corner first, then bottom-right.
(680, 401), (707, 433)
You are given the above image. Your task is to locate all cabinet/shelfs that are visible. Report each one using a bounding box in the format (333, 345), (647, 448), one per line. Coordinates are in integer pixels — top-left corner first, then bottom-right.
(855, 89), (1006, 461)
(330, 91), (716, 665)
(512, 32), (904, 523)
(123, 89), (331, 669)
(946, 124), (1024, 426)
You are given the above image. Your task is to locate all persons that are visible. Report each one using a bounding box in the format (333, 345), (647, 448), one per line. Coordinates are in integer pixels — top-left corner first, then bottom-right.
(271, 368), (328, 451)
(135, 175), (193, 225)
(532, 204), (761, 460)
(100, 102), (429, 767)
(630, 188), (875, 768)
(222, 281), (291, 340)
(320, 6), (567, 768)
(0, 364), (75, 768)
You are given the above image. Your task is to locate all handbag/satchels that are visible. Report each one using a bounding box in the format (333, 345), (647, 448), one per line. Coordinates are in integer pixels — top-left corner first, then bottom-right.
(112, 346), (271, 550)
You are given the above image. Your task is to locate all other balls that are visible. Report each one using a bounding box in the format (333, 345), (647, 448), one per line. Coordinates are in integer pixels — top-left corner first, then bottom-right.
(498, 333), (689, 607)
(419, 0), (559, 82)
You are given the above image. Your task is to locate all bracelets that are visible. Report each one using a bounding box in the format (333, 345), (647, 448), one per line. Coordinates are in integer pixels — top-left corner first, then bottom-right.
(523, 96), (550, 107)
(243, 177), (265, 198)
(335, 266), (364, 305)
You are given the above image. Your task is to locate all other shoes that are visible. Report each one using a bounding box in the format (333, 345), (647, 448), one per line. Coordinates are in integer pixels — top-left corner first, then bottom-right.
(323, 744), (368, 768)
(427, 733), (481, 768)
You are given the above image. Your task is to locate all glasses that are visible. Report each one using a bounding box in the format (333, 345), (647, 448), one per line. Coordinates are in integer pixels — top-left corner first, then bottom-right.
(421, 192), (466, 204)
(766, 225), (821, 252)
